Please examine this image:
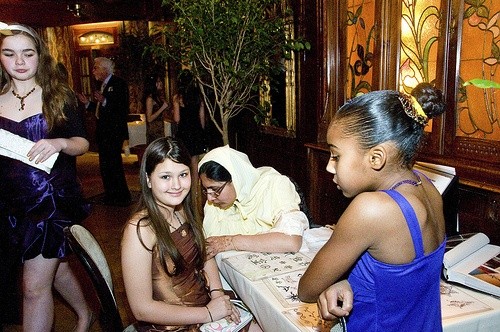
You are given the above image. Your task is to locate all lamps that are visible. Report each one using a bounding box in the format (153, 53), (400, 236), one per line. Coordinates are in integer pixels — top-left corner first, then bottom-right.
(65, 2), (88, 17)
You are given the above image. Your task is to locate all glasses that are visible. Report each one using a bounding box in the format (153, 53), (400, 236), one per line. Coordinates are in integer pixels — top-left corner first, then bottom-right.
(202, 180), (229, 198)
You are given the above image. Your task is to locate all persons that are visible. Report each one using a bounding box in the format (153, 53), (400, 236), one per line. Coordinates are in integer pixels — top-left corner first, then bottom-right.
(198, 146), (307, 332)
(297, 83), (446, 332)
(77, 57), (132, 206)
(120, 136), (265, 332)
(141, 70), (207, 157)
(0, 23), (100, 332)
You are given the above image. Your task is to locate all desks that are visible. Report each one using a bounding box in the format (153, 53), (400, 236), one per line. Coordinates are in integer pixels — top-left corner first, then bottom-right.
(219, 223), (500, 332)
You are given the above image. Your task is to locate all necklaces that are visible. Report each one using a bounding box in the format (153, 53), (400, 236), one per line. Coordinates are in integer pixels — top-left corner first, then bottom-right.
(12, 83), (38, 111)
(168, 213), (188, 237)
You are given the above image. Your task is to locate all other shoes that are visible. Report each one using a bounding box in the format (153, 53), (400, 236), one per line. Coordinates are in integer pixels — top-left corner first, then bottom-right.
(100, 190), (131, 206)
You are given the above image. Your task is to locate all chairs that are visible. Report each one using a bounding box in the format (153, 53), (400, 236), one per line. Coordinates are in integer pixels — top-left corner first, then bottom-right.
(63, 223), (137, 332)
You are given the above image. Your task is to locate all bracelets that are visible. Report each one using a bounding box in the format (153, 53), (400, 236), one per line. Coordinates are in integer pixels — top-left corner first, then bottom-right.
(205, 306), (213, 322)
(231, 234), (241, 251)
(210, 288), (224, 293)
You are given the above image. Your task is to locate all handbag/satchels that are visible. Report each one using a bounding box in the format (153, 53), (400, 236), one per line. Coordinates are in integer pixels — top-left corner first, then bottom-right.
(199, 300), (253, 332)
